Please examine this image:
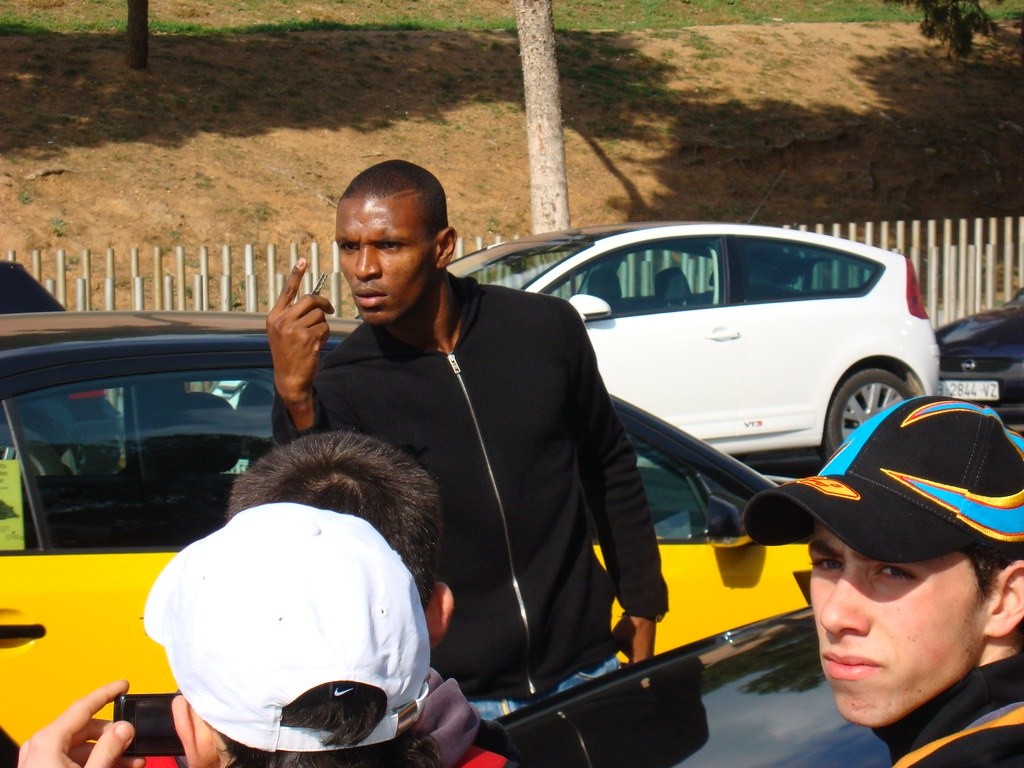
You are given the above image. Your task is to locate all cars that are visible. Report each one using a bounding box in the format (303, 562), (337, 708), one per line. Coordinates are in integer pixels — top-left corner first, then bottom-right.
(463, 590), (919, 766)
(441, 219), (943, 475)
(0, 308), (863, 767)
(928, 290), (1022, 431)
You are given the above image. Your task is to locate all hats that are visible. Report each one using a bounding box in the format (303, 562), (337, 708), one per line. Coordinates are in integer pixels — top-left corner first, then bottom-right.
(742, 387), (1023, 563)
(144, 503), (432, 754)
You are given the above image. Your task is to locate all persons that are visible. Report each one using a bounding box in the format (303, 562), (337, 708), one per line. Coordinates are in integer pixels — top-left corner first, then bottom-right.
(744, 394), (1024, 768)
(15, 430), (515, 768)
(265, 162), (671, 722)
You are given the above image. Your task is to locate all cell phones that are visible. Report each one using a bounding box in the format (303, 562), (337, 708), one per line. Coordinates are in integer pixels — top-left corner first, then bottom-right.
(114, 695), (186, 757)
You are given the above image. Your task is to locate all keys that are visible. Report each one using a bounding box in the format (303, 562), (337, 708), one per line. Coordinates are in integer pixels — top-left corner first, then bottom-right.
(309, 273), (328, 299)
